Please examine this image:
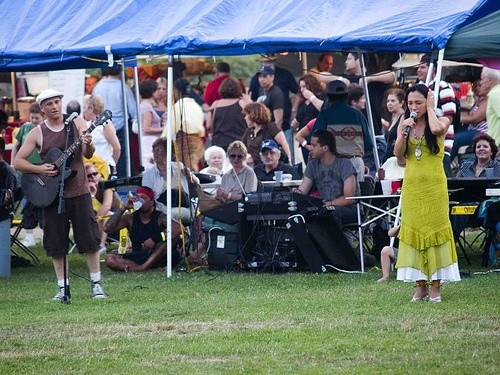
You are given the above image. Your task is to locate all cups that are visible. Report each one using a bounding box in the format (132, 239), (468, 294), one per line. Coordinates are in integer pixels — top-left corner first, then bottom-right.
(133, 197), (145, 210)
(380, 180), (391, 195)
(274, 171), (282, 182)
(485, 168), (493, 178)
(392, 180), (401, 195)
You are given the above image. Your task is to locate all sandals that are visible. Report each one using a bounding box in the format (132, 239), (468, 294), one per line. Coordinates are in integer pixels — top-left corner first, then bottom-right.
(410, 297), (420, 302)
(430, 296), (441, 303)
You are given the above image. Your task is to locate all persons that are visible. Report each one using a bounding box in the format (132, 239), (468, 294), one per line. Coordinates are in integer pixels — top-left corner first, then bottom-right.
(14, 90), (109, 302)
(0, 50), (500, 273)
(392, 83), (462, 305)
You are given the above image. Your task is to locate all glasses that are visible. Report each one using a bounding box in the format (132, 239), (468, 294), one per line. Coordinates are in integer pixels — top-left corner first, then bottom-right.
(87, 171), (98, 179)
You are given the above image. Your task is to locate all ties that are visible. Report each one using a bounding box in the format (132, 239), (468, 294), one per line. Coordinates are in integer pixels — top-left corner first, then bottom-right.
(229, 154), (245, 159)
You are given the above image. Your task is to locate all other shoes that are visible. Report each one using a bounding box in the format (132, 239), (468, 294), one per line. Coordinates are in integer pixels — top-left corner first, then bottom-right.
(22, 237), (35, 246)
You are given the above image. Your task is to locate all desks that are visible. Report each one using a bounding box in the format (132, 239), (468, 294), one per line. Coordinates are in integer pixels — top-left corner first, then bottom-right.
(346, 195), (401, 273)
(447, 177), (500, 262)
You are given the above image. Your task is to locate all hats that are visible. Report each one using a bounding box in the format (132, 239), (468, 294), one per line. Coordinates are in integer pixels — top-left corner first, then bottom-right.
(326, 80), (348, 94)
(260, 139), (279, 153)
(259, 53), (280, 62)
(36, 89), (64, 106)
(257, 65), (274, 75)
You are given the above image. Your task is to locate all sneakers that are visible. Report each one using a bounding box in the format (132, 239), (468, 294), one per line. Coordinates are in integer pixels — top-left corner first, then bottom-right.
(53, 285), (71, 302)
(91, 280), (106, 299)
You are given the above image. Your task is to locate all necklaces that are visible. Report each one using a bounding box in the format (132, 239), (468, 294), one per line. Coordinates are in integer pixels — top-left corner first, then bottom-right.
(412, 123), (425, 159)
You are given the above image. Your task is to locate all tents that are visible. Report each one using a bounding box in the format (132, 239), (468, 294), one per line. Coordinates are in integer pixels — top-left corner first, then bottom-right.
(1, 54), (143, 178)
(390, 10), (499, 247)
(2, 0), (499, 277)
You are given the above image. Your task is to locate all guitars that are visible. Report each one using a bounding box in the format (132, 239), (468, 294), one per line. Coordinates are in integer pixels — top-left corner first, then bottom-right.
(20, 109), (114, 209)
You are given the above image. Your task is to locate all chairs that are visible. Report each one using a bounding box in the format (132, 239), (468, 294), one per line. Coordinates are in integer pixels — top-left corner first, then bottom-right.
(10, 188), (42, 267)
(344, 176), (376, 249)
(481, 202), (500, 267)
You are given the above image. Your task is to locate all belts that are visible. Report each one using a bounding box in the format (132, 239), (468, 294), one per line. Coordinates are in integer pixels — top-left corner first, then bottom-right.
(345, 155), (361, 158)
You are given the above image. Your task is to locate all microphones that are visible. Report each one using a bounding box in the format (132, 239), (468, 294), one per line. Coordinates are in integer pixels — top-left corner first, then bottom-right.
(404, 111), (418, 135)
(65, 111), (78, 125)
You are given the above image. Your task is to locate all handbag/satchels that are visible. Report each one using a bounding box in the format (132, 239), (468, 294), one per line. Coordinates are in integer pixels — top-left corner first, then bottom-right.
(0, 187), (14, 215)
